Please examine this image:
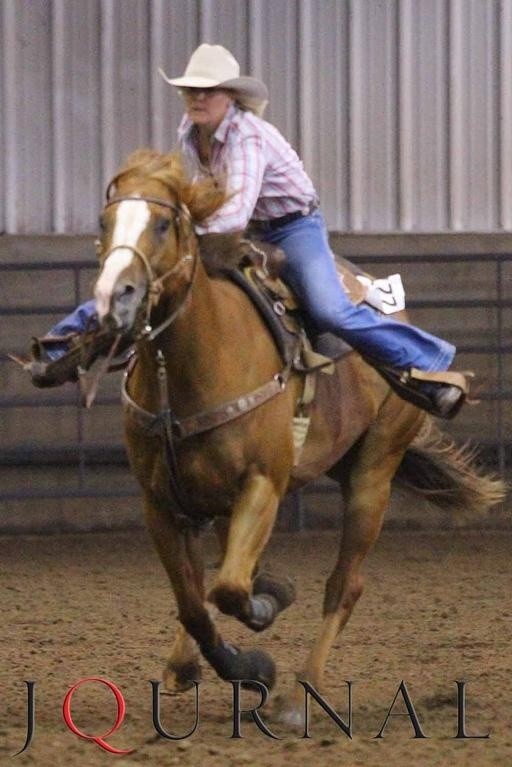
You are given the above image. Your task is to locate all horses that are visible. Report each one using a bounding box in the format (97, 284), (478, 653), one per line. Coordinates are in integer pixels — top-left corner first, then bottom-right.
(92, 145), (506, 726)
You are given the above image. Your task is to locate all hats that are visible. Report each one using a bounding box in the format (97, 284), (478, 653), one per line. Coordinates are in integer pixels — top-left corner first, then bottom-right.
(157, 43), (269, 116)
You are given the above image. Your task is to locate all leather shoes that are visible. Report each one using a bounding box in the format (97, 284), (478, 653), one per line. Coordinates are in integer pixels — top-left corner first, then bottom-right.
(30, 353), (62, 388)
(431, 381), (465, 419)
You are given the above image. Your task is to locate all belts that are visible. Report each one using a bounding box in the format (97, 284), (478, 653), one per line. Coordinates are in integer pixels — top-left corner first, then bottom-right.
(250, 199), (319, 232)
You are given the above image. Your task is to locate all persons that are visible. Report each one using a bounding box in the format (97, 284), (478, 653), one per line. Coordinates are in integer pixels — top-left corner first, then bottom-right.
(30, 45), (464, 415)
